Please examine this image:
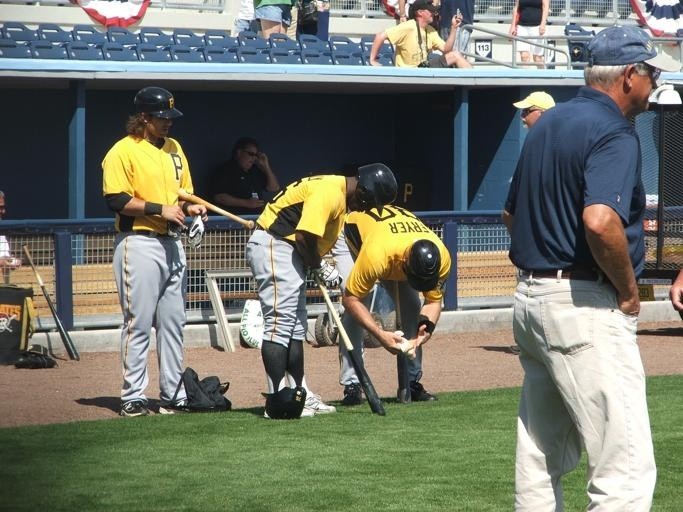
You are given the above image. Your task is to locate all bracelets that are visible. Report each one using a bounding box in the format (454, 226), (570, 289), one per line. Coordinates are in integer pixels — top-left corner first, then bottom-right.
(415, 319), (437, 336)
(182, 201), (192, 218)
(145, 201), (163, 218)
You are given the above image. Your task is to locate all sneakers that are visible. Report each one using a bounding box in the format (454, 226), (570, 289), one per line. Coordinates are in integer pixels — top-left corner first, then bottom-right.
(397, 382), (436, 401)
(302, 394), (336, 417)
(120, 401), (148, 417)
(159, 399), (189, 414)
(342, 383), (364, 405)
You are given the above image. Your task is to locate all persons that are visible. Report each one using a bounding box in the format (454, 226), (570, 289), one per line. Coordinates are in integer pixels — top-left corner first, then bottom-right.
(369, 0), (475, 67)
(210, 137), (281, 215)
(669, 265), (683, 318)
(94, 88), (209, 418)
(499, 26), (657, 512)
(328, 203), (452, 407)
(1, 191), (23, 282)
(230, 0), (332, 43)
(508, 1), (551, 70)
(510, 83), (556, 359)
(244, 162), (398, 417)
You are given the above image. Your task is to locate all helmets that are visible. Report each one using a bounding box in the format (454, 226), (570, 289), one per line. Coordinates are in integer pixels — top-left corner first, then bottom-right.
(357, 163), (398, 217)
(261, 386), (306, 419)
(402, 239), (440, 291)
(134, 87), (184, 121)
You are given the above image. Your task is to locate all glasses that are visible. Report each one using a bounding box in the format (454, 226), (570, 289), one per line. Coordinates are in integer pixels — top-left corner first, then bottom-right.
(243, 150), (257, 156)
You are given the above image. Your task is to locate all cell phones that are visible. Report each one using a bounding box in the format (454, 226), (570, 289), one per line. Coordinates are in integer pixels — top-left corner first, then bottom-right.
(457, 9), (460, 18)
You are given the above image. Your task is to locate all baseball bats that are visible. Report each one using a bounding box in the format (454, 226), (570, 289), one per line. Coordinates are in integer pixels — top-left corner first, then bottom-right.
(393, 280), (410, 404)
(179, 188), (255, 228)
(312, 267), (384, 416)
(24, 244), (79, 361)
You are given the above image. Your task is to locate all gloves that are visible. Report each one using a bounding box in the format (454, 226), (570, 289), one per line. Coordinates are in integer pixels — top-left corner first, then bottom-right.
(306, 259), (342, 287)
(187, 210), (204, 249)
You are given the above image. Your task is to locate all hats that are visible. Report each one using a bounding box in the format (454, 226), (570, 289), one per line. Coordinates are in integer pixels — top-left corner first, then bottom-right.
(583, 25), (682, 72)
(412, 0), (436, 11)
(512, 91), (556, 110)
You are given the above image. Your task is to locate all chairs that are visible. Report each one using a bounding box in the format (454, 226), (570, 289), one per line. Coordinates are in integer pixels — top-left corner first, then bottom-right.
(563, 23), (598, 69)
(0, 23), (443, 68)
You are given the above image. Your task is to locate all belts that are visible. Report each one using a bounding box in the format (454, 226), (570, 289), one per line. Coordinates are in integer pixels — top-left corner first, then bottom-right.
(137, 229), (167, 237)
(522, 269), (611, 283)
